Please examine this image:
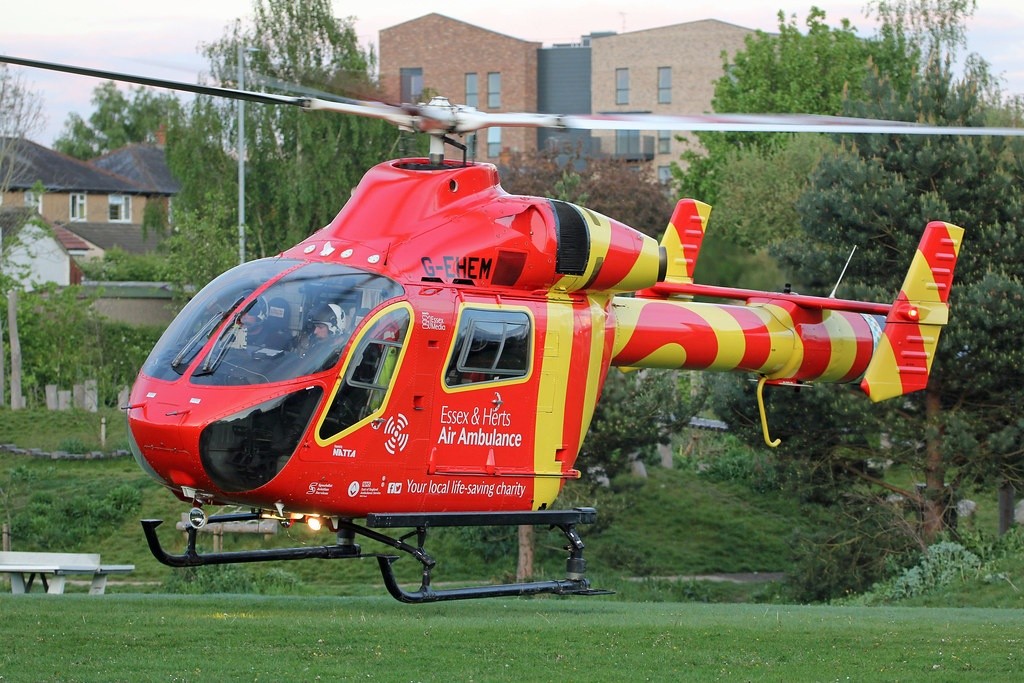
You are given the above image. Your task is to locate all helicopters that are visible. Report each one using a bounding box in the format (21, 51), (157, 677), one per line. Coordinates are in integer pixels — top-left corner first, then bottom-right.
(1, 55), (1024, 604)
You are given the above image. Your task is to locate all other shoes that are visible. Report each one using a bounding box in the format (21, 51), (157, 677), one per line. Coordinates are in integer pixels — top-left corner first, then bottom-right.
(270, 424), (303, 455)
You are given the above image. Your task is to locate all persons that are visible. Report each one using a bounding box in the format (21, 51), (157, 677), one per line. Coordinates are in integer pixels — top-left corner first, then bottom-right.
(296, 303), (347, 362)
(234, 290), (282, 350)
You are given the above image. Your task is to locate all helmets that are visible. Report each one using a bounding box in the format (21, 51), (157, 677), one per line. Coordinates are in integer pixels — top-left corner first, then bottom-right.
(308, 304), (346, 338)
(235, 294), (268, 327)
